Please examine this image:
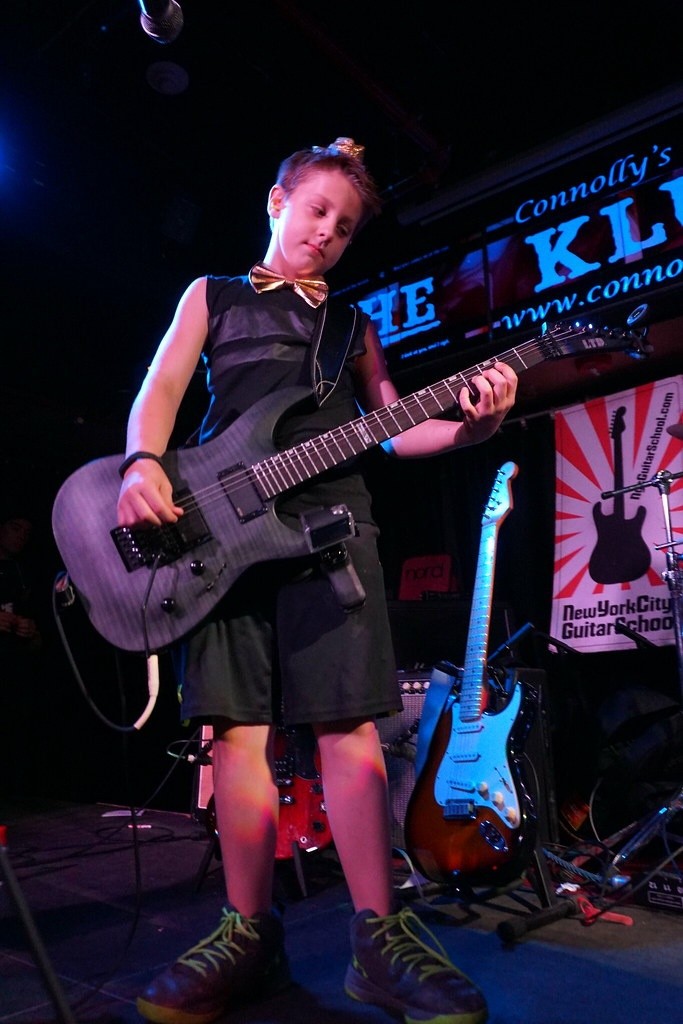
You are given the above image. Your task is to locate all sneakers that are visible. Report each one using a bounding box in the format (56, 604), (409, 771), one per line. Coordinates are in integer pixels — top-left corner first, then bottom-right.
(136, 902), (289, 1024)
(344, 899), (488, 1024)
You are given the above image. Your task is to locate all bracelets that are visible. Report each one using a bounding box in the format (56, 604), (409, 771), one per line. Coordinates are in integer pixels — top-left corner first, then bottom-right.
(118, 452), (166, 479)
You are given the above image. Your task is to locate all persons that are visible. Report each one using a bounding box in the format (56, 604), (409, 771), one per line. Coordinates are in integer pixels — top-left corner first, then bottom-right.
(136, 137), (518, 1024)
(0, 510), (42, 794)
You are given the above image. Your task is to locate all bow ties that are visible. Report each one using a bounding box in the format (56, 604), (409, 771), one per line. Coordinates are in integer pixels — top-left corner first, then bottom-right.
(248, 260), (328, 309)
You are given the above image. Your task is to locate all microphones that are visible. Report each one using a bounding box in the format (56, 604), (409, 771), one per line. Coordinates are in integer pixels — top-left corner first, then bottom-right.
(183, 754), (212, 766)
(380, 741), (417, 762)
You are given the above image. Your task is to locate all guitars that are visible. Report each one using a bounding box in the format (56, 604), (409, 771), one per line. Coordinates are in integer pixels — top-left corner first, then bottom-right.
(204, 724), (335, 869)
(44, 310), (649, 666)
(399, 457), (544, 905)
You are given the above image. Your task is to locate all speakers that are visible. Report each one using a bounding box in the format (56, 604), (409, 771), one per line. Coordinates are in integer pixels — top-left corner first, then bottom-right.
(192, 723), (216, 812)
(374, 668), (560, 857)
